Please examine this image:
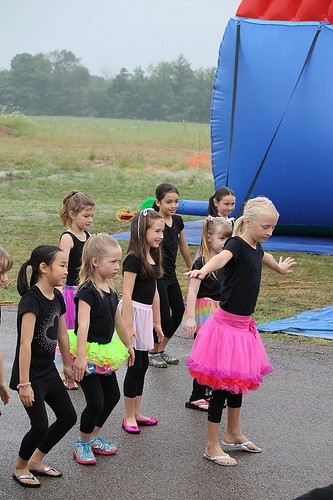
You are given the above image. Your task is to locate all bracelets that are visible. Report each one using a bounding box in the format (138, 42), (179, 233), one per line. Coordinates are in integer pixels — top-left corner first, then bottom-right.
(17, 381), (31, 389)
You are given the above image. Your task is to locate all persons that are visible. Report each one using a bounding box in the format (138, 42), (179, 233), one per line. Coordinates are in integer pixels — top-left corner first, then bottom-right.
(150, 184), (237, 412)
(116, 208), (165, 432)
(0, 246), (78, 489)
(55, 191), (96, 390)
(72, 232), (134, 465)
(182, 195), (297, 467)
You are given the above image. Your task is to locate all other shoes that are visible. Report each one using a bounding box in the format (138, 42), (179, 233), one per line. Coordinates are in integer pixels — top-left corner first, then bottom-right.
(135, 417), (158, 425)
(122, 418), (140, 433)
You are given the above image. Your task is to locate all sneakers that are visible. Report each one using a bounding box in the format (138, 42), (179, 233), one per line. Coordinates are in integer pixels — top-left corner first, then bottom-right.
(160, 352), (179, 363)
(147, 352), (168, 367)
(88, 435), (118, 455)
(71, 439), (97, 465)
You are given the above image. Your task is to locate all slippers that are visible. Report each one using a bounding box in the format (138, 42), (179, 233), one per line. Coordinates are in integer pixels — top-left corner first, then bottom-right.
(13, 469), (41, 488)
(204, 448), (238, 466)
(185, 399), (210, 411)
(222, 437), (262, 452)
(61, 378), (79, 389)
(28, 466), (62, 477)
